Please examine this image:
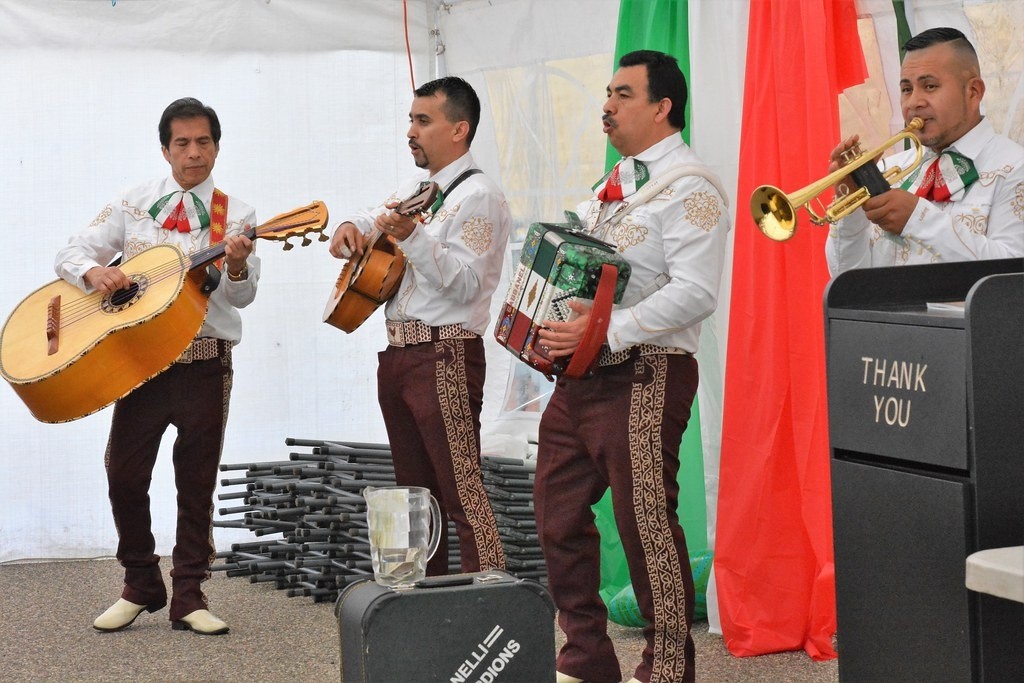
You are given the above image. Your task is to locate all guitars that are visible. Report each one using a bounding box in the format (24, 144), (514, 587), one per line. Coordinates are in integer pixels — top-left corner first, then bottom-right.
(0, 199), (332, 426)
(327, 182), (446, 333)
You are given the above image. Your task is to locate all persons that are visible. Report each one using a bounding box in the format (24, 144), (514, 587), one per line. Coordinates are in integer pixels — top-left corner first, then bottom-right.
(823, 25), (1022, 276)
(534, 49), (729, 683)
(55, 91), (259, 639)
(329, 73), (507, 572)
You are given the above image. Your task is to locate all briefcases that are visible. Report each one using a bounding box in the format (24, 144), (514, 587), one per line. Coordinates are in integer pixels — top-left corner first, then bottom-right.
(334, 569), (557, 683)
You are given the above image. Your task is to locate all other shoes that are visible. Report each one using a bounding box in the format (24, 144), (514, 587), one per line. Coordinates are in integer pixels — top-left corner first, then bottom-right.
(627, 678), (642, 683)
(556, 671), (584, 683)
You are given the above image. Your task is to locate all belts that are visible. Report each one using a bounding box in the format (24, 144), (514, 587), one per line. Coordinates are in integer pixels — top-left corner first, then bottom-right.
(385, 320), (477, 347)
(597, 343), (686, 367)
(175, 338), (233, 364)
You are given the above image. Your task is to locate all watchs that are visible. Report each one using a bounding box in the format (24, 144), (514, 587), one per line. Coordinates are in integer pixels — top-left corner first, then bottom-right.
(226, 263), (249, 282)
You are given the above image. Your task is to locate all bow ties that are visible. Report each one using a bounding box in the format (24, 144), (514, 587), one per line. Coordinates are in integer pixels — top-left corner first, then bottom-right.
(898, 150), (979, 203)
(590, 157), (650, 203)
(405, 181), (443, 224)
(148, 191), (210, 239)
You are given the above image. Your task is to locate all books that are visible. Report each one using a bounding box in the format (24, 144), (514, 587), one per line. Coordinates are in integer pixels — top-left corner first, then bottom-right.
(926, 301), (965, 313)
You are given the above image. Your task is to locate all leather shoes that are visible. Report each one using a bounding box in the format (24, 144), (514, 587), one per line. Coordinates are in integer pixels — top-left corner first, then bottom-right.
(93, 597), (167, 631)
(172, 609), (229, 634)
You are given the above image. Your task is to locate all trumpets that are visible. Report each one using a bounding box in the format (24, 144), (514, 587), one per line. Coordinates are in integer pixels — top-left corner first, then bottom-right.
(749, 112), (928, 243)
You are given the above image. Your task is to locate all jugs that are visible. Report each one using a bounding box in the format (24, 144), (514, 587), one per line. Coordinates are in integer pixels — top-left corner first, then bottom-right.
(362, 484), (442, 588)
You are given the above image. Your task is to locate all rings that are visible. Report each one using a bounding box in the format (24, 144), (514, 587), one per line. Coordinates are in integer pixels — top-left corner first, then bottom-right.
(390, 225), (394, 229)
